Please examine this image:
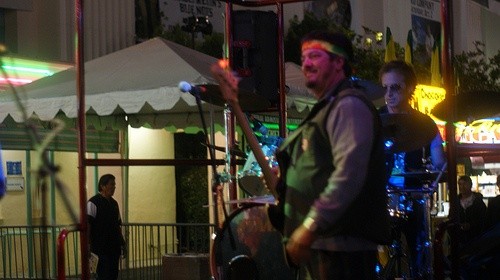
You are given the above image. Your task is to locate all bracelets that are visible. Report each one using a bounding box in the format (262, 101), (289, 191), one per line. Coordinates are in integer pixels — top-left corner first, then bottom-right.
(303, 217), (319, 234)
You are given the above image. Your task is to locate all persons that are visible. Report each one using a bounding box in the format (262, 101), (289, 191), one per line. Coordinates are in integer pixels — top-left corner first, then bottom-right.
(448, 175), (500, 280)
(377, 59), (445, 280)
(426, 24), (434, 64)
(88, 174), (127, 280)
(283, 31), (379, 280)
(413, 21), (422, 69)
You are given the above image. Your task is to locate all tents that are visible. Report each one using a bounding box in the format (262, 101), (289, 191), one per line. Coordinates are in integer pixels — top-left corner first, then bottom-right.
(0, 36), (318, 130)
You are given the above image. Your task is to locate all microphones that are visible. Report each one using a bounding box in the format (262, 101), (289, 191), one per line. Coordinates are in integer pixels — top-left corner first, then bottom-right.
(178, 81), (229, 108)
(248, 112), (269, 135)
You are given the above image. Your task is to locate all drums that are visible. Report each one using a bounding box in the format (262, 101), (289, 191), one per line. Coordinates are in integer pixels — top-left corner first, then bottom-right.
(383, 190), (413, 222)
(239, 137), (284, 197)
(211, 202), (307, 280)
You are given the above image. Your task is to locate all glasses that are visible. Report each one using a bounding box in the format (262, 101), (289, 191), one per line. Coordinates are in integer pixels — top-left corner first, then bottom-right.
(382, 83), (409, 94)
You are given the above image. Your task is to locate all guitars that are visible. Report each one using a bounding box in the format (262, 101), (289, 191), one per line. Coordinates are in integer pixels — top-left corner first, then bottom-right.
(209, 58), (293, 271)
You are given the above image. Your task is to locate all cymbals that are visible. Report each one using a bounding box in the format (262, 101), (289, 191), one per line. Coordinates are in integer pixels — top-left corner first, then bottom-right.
(204, 143), (249, 158)
(394, 168), (451, 183)
(431, 89), (500, 122)
(378, 113), (439, 152)
(188, 83), (228, 107)
